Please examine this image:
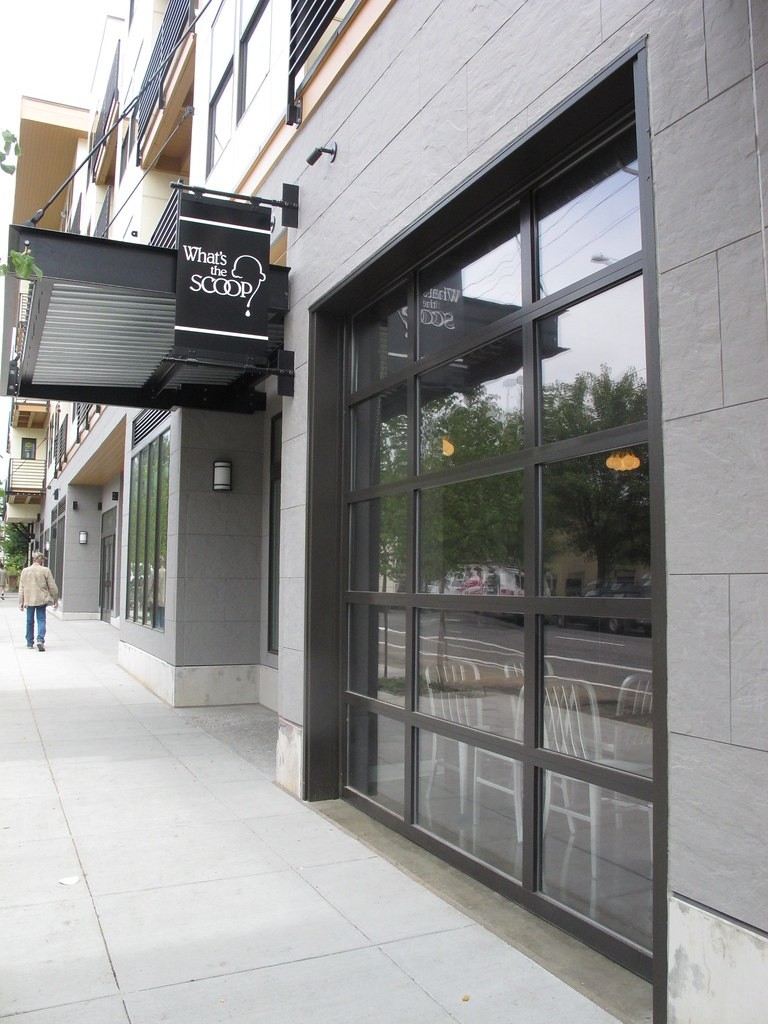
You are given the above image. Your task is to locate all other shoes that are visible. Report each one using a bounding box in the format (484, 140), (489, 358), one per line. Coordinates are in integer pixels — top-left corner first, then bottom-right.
(27, 644), (33, 649)
(1, 596), (4, 600)
(36, 641), (45, 651)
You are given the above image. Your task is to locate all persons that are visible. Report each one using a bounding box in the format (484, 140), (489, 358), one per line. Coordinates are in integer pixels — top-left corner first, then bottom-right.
(148, 556), (165, 628)
(19, 552), (58, 651)
(0, 565), (8, 599)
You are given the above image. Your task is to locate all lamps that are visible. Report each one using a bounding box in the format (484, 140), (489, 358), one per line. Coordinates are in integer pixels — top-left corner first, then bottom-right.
(79, 531), (88, 544)
(98, 502), (102, 510)
(212, 460), (231, 493)
(112, 491), (118, 501)
(444, 429), (454, 455)
(73, 501), (78, 510)
(306, 142), (337, 166)
(605, 448), (641, 472)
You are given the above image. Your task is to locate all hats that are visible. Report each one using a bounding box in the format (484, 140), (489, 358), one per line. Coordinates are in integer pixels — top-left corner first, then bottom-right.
(33, 552), (46, 559)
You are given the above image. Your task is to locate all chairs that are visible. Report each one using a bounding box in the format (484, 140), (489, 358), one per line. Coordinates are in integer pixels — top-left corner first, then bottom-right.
(423, 654), (652, 880)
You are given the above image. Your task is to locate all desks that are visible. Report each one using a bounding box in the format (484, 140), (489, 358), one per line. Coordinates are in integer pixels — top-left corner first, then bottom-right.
(429, 677), (652, 841)
